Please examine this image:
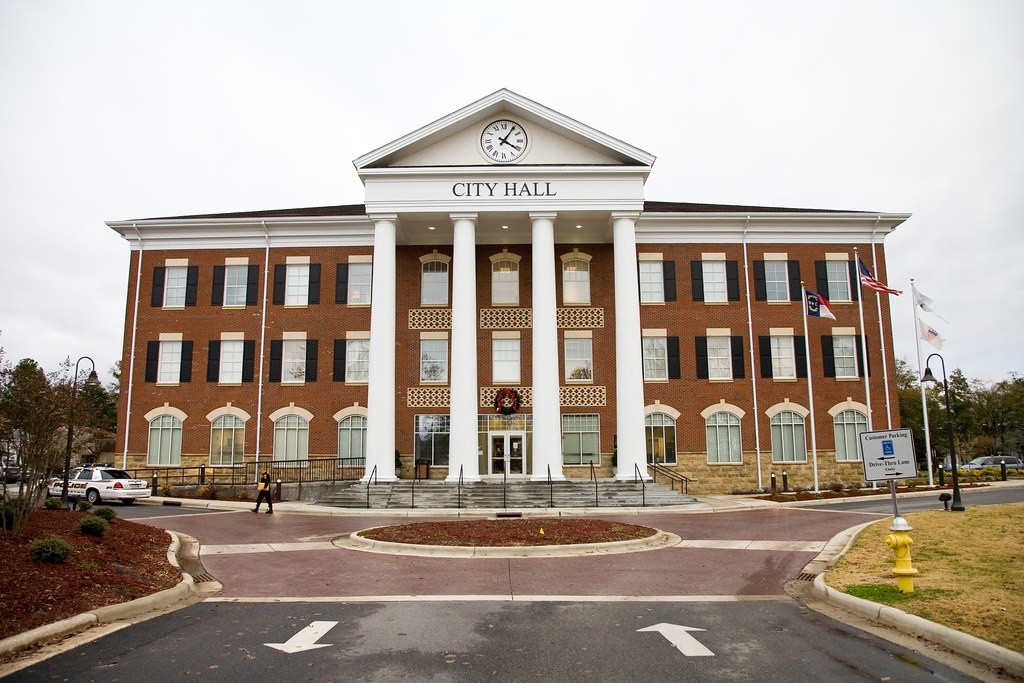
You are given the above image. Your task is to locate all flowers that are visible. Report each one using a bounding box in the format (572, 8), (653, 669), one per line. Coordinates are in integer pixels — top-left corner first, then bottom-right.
(494, 389), (521, 416)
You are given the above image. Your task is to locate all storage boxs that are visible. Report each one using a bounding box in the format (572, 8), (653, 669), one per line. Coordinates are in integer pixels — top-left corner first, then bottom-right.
(416, 463), (428, 479)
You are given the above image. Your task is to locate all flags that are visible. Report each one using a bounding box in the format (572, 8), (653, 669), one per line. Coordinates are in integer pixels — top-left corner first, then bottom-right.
(857, 252), (903, 295)
(913, 286), (950, 323)
(918, 318), (946, 349)
(804, 286), (837, 321)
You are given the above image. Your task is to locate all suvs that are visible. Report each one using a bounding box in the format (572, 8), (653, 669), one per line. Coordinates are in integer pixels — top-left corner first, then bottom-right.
(960, 455), (1024, 473)
(0, 458), (20, 484)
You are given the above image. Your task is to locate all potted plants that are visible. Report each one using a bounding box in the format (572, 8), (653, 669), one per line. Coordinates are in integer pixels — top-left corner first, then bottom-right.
(611, 449), (617, 475)
(395, 449), (402, 477)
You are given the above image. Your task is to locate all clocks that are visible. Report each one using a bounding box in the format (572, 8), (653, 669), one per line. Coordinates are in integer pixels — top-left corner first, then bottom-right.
(476, 114), (532, 165)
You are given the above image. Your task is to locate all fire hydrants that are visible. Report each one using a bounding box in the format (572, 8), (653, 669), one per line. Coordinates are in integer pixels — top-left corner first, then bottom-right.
(885, 514), (918, 594)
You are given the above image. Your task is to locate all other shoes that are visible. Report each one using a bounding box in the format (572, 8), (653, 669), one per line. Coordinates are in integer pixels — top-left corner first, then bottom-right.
(250, 509), (257, 513)
(265, 509), (273, 514)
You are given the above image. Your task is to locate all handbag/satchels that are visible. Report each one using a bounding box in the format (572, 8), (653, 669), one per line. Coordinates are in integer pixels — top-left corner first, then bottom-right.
(257, 482), (270, 491)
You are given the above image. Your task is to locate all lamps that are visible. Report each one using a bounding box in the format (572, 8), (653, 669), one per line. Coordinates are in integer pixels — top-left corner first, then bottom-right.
(782, 470), (787, 475)
(201, 463), (205, 468)
(153, 473), (157, 477)
(770, 471), (775, 477)
(276, 478), (281, 482)
(938, 463), (943, 468)
(1000, 458), (1005, 464)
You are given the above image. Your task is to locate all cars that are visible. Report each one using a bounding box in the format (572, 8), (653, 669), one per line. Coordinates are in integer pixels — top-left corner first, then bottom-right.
(19, 463), (46, 480)
(37, 463), (152, 506)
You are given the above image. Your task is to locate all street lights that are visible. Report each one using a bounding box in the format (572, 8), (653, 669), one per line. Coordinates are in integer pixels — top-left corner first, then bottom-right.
(59, 356), (100, 513)
(921, 353), (966, 512)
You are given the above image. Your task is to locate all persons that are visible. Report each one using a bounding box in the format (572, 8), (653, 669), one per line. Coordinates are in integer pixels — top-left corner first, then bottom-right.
(251, 469), (273, 514)
